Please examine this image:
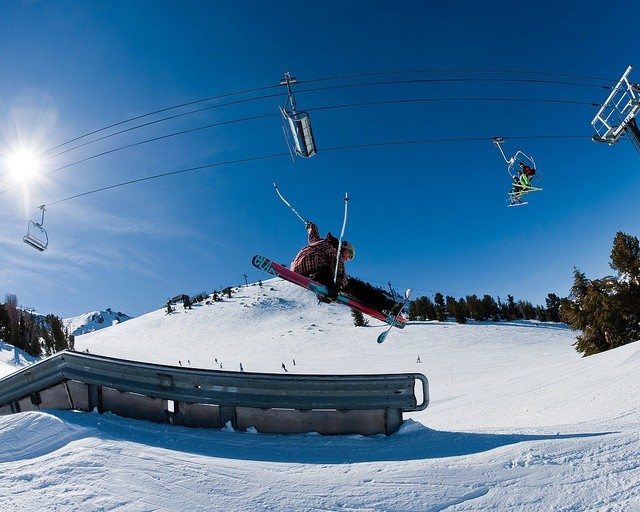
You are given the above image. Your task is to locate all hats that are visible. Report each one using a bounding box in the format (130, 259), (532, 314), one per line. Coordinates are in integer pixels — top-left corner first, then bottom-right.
(342, 240), (355, 262)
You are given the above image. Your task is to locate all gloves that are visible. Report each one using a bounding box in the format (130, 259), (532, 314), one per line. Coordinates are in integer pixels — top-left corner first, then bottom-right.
(327, 283), (343, 303)
(305, 219), (311, 229)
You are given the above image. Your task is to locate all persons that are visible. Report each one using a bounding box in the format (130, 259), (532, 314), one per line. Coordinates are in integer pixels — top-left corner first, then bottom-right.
(510, 162), (533, 205)
(291, 221), (355, 304)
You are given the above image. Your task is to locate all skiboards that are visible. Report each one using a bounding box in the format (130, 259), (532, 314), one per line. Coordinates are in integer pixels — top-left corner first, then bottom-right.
(252, 255), (407, 329)
(507, 183), (543, 195)
(509, 202), (528, 206)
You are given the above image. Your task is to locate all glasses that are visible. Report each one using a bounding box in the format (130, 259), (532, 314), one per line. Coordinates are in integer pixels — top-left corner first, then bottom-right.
(348, 249), (353, 260)
